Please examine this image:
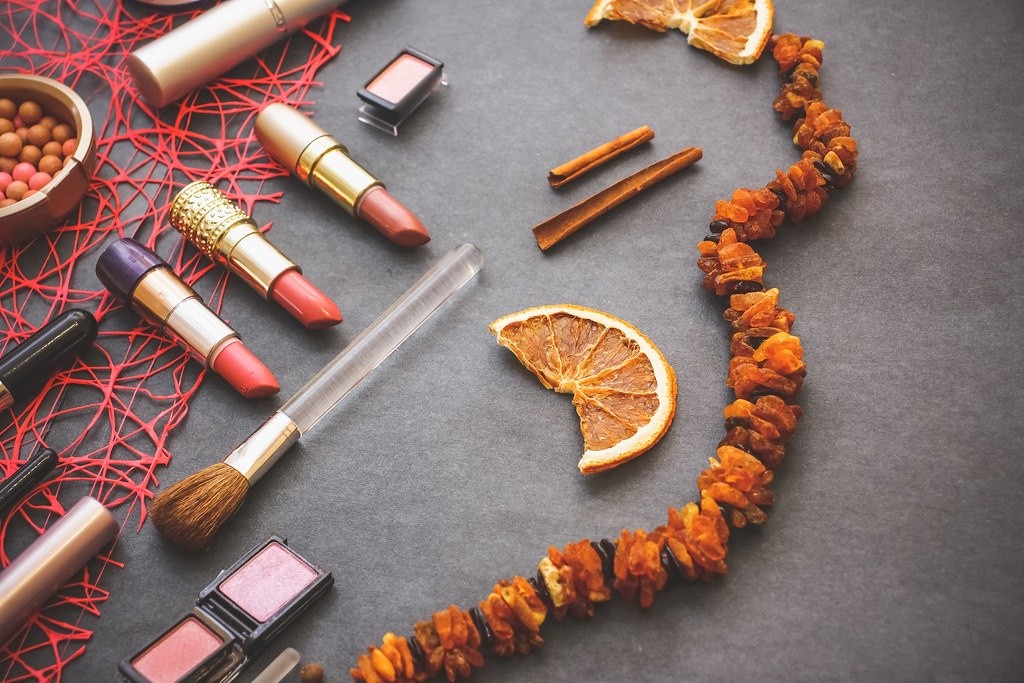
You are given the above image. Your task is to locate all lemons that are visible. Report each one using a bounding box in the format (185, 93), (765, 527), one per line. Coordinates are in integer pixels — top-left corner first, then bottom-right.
(487, 302), (677, 476)
(584, 0), (775, 64)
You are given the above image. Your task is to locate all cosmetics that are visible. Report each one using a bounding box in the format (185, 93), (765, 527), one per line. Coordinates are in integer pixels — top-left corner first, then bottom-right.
(0, 72), (96, 241)
(118, 532), (336, 683)
(356, 45), (449, 137)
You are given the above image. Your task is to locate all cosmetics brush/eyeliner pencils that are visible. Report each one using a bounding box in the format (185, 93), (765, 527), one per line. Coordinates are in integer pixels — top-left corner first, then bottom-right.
(0, 448), (59, 508)
(151, 242), (484, 555)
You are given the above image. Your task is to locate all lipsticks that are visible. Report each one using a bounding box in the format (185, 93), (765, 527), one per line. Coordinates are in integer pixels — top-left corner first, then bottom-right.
(254, 103), (430, 247)
(167, 181), (345, 332)
(95, 237), (281, 402)
(2, 497), (120, 642)
(126, 0), (345, 112)
(1, 306), (97, 419)
